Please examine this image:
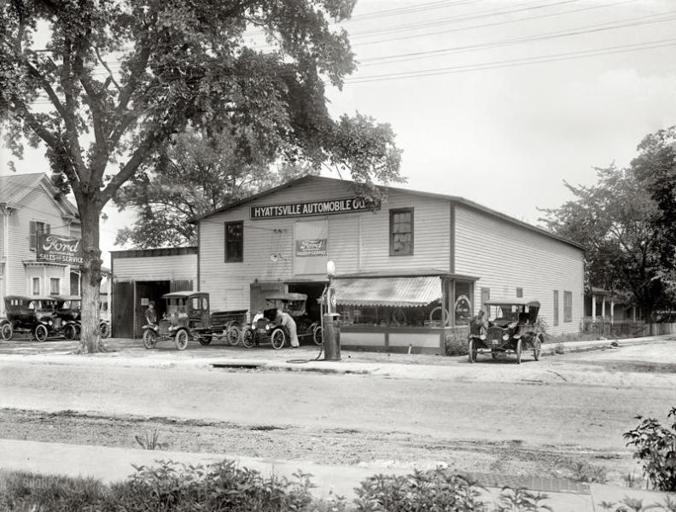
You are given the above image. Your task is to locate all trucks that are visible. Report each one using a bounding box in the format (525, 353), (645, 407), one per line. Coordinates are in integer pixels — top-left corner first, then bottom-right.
(139, 290), (249, 352)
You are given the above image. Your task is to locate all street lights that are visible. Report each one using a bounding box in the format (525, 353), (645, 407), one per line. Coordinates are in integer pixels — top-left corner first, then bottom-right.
(324, 258), (336, 313)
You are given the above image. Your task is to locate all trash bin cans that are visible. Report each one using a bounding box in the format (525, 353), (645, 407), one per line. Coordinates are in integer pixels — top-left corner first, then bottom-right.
(323, 312), (341, 361)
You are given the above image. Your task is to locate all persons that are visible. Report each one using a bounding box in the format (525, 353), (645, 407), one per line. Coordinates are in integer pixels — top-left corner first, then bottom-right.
(470, 311), (487, 335)
(252, 309), (264, 346)
(276, 309), (300, 348)
(146, 301), (157, 326)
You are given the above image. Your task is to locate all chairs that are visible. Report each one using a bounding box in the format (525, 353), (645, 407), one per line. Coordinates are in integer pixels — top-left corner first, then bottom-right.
(502, 312), (534, 334)
(283, 303), (307, 332)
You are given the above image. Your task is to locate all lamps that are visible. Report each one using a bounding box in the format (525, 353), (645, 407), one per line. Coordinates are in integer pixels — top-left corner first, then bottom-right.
(268, 252), (287, 264)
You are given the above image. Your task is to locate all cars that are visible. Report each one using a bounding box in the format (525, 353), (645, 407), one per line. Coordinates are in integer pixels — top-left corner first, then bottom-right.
(467, 294), (543, 364)
(47, 294), (110, 339)
(241, 292), (326, 349)
(0, 294), (75, 343)
